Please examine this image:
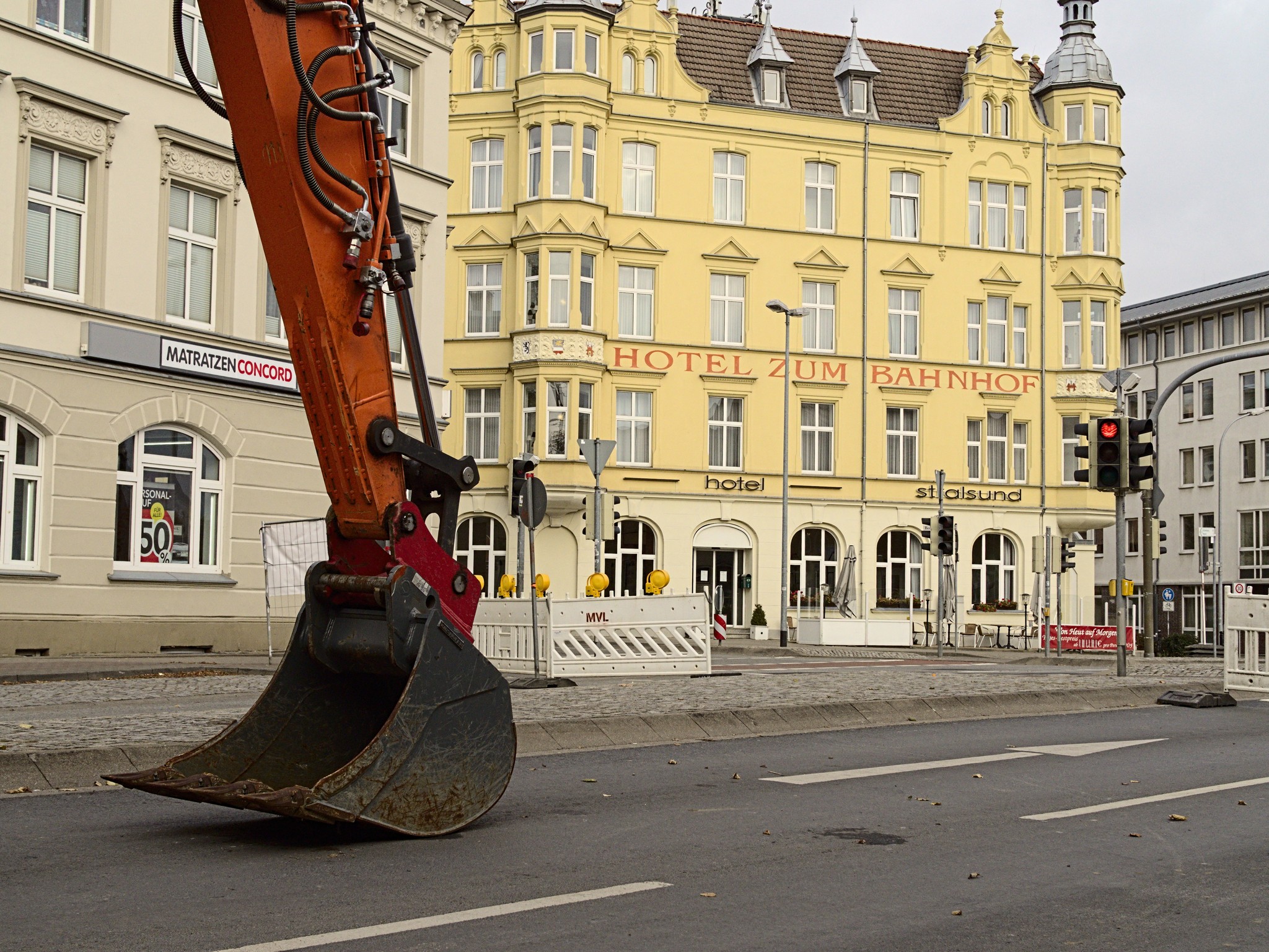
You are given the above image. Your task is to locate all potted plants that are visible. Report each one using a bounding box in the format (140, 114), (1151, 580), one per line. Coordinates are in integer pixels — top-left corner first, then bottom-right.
(749, 604), (769, 640)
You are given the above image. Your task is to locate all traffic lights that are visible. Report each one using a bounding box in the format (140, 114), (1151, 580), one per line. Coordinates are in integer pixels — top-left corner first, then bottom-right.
(937, 516), (953, 555)
(1051, 535), (1069, 574)
(1151, 520), (1167, 558)
(1097, 417), (1124, 489)
(1066, 541), (1076, 572)
(1138, 424), (1158, 490)
(507, 459), (538, 517)
(1074, 420), (1096, 489)
(921, 517), (940, 556)
(601, 495), (620, 540)
(1122, 417), (1154, 489)
(582, 494), (596, 540)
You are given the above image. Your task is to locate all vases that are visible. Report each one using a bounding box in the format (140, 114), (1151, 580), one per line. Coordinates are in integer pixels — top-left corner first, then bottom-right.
(973, 603), (996, 610)
(790, 601), (816, 607)
(876, 602), (899, 608)
(899, 603), (921, 608)
(816, 602), (837, 607)
(995, 604), (1016, 610)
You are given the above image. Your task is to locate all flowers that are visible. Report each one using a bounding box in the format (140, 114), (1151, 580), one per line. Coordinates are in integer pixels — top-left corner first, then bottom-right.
(814, 593), (833, 602)
(877, 594), (899, 603)
(976, 602), (997, 612)
(995, 598), (1018, 607)
(899, 595), (923, 604)
(790, 591), (815, 602)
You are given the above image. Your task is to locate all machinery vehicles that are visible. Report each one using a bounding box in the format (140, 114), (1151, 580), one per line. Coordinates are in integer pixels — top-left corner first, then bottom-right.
(100, 0), (517, 838)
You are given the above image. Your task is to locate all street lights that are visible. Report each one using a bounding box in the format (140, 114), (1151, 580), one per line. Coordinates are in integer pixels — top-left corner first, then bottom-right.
(766, 299), (809, 647)
(1219, 407), (1266, 644)
(1019, 593), (1032, 650)
(922, 589), (934, 647)
(820, 583), (832, 619)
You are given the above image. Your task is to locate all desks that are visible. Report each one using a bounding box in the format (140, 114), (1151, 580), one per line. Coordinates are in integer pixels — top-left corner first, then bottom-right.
(982, 624), (1028, 648)
(932, 622), (968, 647)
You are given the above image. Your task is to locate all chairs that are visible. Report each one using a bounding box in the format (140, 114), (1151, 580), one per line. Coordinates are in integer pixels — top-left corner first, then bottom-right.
(912, 622), (1038, 649)
(787, 617), (797, 642)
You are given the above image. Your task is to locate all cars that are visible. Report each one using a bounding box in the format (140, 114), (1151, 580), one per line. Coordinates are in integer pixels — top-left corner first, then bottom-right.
(172, 542), (190, 551)
